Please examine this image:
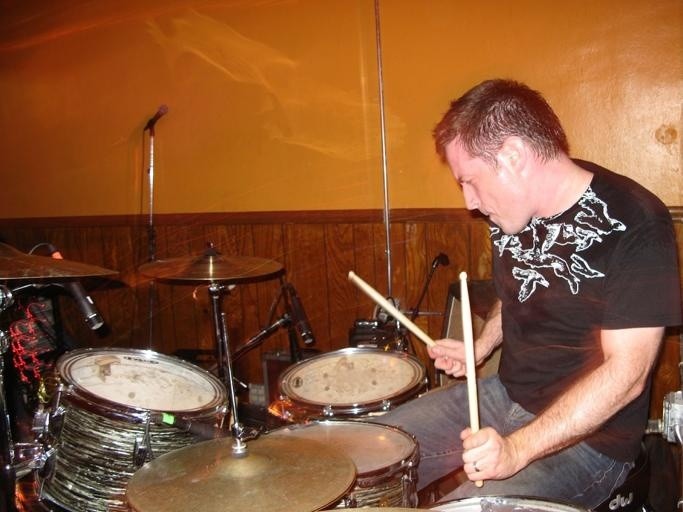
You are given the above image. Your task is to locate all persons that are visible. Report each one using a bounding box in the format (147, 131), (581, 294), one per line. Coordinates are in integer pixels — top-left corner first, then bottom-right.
(361, 78), (683, 512)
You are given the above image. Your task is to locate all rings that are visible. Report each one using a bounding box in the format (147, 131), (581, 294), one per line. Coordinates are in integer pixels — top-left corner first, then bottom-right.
(473, 462), (479, 472)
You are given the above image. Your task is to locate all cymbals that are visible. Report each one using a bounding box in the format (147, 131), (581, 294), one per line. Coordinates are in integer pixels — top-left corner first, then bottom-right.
(125, 434), (357, 511)
(0, 241), (120, 281)
(139, 254), (282, 280)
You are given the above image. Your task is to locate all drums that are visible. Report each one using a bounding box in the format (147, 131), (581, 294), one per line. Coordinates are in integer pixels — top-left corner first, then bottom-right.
(259, 420), (420, 509)
(33, 349), (228, 512)
(429, 495), (589, 512)
(278, 348), (428, 420)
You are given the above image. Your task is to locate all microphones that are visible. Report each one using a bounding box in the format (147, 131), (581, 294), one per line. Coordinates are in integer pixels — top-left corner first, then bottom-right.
(143, 104), (169, 130)
(288, 283), (313, 344)
(48, 243), (104, 330)
